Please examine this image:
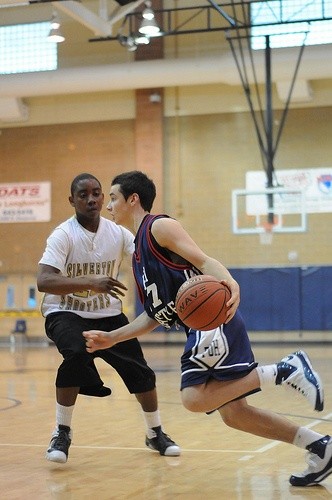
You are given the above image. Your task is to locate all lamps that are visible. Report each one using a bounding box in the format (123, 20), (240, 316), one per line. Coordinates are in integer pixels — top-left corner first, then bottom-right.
(48, 14), (65, 43)
(126, 0), (160, 50)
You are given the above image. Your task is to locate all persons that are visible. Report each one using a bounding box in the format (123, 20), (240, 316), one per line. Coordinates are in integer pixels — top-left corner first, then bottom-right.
(36, 173), (181, 464)
(82, 169), (332, 487)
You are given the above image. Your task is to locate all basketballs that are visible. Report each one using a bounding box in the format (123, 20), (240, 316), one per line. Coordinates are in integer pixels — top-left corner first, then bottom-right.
(176, 275), (235, 331)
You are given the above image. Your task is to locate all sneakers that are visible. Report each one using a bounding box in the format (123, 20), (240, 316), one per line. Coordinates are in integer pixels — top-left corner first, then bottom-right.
(46, 424), (73, 463)
(145, 425), (181, 456)
(289, 435), (332, 486)
(275, 349), (324, 411)
